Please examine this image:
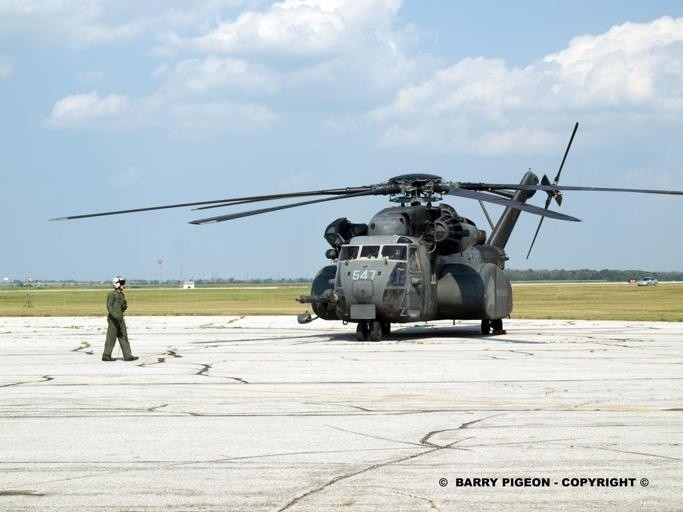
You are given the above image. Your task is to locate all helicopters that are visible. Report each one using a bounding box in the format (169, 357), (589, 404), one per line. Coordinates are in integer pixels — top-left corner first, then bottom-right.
(47, 120), (682, 342)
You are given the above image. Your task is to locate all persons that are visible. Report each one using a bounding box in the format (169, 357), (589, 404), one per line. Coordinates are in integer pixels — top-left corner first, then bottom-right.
(102, 275), (139, 361)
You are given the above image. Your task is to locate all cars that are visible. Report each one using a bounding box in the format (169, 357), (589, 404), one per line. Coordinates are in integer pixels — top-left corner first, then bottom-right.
(637, 277), (658, 287)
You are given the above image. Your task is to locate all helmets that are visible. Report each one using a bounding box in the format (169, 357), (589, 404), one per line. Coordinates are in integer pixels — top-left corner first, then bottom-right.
(112, 275), (125, 288)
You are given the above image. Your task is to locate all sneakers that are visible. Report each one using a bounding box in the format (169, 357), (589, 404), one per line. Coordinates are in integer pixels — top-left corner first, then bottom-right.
(124, 355), (139, 361)
(101, 356), (117, 361)
